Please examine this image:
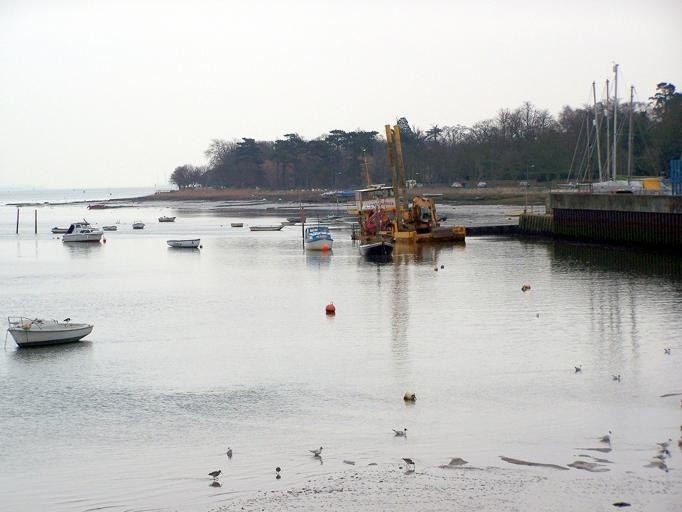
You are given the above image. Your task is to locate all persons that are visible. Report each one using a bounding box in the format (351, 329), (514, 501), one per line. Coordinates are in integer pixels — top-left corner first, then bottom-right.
(385, 217), (392, 231)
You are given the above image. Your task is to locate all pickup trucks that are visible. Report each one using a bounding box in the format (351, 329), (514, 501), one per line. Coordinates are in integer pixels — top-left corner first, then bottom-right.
(557, 182), (580, 188)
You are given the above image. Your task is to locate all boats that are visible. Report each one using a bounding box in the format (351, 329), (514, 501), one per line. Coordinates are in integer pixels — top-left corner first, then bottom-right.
(249, 225), (285, 232)
(346, 149), (409, 219)
(283, 214), (346, 250)
(133, 222), (145, 229)
(62, 218), (106, 244)
(166, 238), (203, 250)
(52, 227), (69, 234)
(103, 225), (118, 231)
(358, 237), (395, 263)
(231, 223), (244, 228)
(158, 216), (177, 222)
(4, 316), (94, 350)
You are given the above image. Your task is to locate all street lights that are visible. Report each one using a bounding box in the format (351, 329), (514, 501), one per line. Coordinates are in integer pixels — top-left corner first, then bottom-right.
(527, 160), (536, 213)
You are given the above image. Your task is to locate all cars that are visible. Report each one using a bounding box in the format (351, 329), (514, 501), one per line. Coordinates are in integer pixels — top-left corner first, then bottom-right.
(477, 181), (489, 188)
(519, 180), (529, 186)
(450, 182), (466, 187)
